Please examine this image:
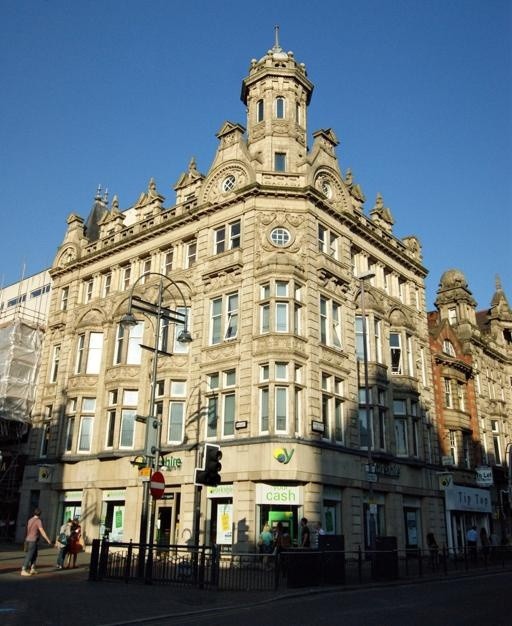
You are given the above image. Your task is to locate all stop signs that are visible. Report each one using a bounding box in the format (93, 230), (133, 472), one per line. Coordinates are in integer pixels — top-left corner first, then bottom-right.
(150, 472), (166, 499)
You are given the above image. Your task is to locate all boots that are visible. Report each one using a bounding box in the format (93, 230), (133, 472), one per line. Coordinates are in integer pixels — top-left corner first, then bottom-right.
(21, 564), (39, 576)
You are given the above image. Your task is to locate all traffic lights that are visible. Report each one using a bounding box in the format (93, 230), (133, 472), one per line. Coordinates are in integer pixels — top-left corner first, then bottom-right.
(194, 443), (222, 487)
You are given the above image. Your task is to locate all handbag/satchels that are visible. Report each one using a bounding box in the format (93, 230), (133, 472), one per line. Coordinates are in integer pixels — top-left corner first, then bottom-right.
(58, 532), (68, 545)
(257, 539), (263, 547)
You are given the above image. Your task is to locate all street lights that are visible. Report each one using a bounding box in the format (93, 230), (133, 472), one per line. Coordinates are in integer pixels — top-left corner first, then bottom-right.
(358, 272), (375, 551)
(118, 272), (193, 584)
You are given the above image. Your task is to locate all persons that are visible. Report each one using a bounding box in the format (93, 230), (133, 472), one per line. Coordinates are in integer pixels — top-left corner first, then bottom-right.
(272, 532), (295, 554)
(20, 507), (53, 578)
(56, 518), (74, 571)
(315, 520), (326, 549)
(426, 527), (442, 570)
(489, 530), (501, 563)
(300, 517), (311, 553)
(64, 518), (85, 570)
(480, 527), (491, 565)
(466, 525), (478, 563)
(258, 524), (274, 567)
(274, 522), (284, 541)
